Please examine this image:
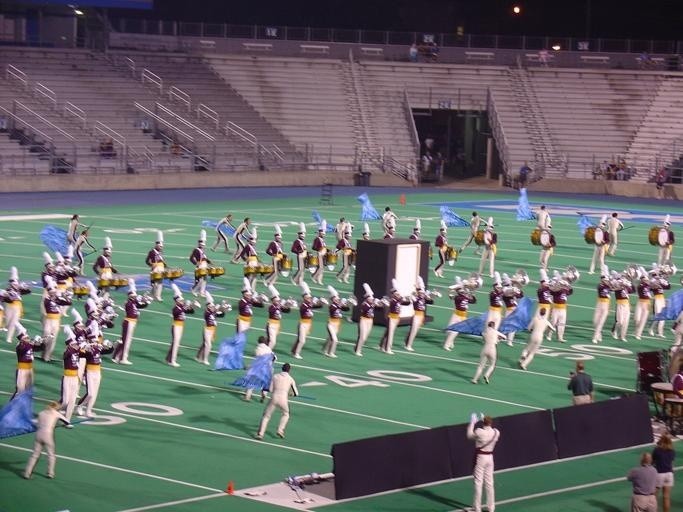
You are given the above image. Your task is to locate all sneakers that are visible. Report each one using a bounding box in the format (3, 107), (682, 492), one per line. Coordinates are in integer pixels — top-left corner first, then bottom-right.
(471, 379), (478, 384)
(520, 355), (526, 361)
(520, 364), (527, 370)
(48, 474), (57, 479)
(240, 394), (251, 402)
(58, 405), (96, 430)
(484, 376), (489, 385)
(111, 357), (181, 368)
(254, 433), (264, 440)
(276, 431), (285, 439)
(24, 474), (34, 480)
(591, 327), (666, 344)
(257, 396), (266, 404)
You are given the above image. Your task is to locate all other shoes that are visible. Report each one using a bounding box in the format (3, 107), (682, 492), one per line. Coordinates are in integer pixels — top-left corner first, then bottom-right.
(443, 344), (455, 351)
(546, 335), (550, 341)
(558, 338), (567, 343)
(506, 341), (513, 347)
(193, 343), (416, 366)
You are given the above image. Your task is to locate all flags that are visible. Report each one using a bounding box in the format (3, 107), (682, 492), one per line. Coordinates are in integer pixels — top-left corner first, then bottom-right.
(358, 192), (381, 220)
(517, 184), (534, 221)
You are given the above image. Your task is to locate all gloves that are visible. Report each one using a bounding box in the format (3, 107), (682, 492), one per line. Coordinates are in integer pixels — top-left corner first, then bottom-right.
(470, 413), (479, 424)
(481, 413), (486, 422)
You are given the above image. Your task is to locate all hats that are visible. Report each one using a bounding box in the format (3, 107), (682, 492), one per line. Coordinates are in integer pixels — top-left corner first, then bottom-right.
(241, 275), (429, 303)
(544, 224), (553, 230)
(127, 229), (217, 309)
(8, 234), (115, 347)
(248, 220), (449, 243)
(486, 224), (494, 230)
(600, 268), (664, 283)
(663, 221), (671, 227)
(455, 272), (571, 294)
(598, 222), (606, 228)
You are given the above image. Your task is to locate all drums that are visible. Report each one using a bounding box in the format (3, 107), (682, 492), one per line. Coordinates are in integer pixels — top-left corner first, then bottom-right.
(475, 229), (493, 245)
(531, 229), (551, 246)
(444, 246), (457, 261)
(649, 226), (669, 247)
(584, 227), (603, 245)
(429, 245), (433, 259)
(72, 249), (356, 298)
(650, 382), (683, 419)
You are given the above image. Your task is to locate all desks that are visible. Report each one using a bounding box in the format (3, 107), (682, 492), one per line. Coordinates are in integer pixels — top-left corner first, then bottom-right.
(662, 398), (683, 438)
(651, 383), (673, 418)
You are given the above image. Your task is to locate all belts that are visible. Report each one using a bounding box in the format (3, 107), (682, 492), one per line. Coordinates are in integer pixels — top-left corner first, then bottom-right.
(574, 393), (590, 396)
(476, 450), (493, 454)
(634, 493), (655, 496)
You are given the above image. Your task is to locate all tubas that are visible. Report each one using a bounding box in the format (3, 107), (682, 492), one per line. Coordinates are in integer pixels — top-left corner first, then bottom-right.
(449, 265), (580, 291)
(606, 260), (678, 291)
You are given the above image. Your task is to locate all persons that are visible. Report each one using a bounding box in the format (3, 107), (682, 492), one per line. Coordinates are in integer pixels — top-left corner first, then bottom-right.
(652, 435), (676, 512)
(409, 41), (441, 62)
(0, 204), (683, 438)
(601, 160), (629, 181)
(23, 402), (71, 479)
(467, 411), (500, 512)
(629, 452), (660, 512)
(520, 161), (531, 182)
(538, 48), (549, 67)
(422, 152), (444, 180)
(656, 167), (669, 183)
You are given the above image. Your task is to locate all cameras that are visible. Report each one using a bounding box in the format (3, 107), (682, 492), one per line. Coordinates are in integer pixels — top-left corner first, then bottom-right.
(470, 413), (484, 423)
